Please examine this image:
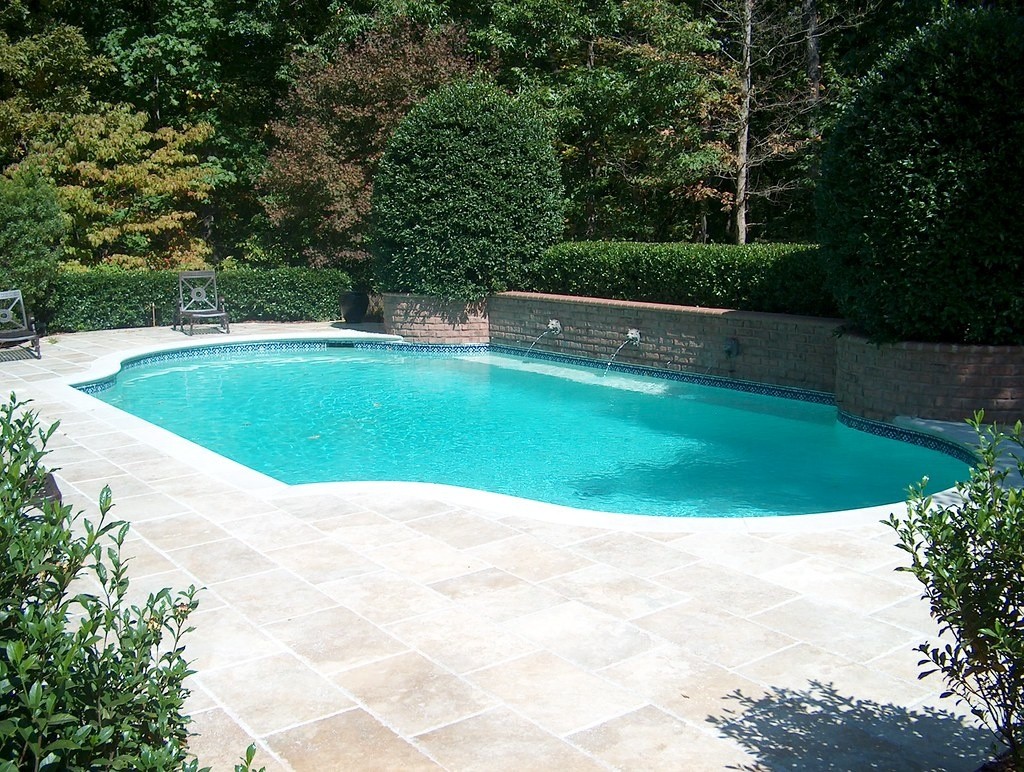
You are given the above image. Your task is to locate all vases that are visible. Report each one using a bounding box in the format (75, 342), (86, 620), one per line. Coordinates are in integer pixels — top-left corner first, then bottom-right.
(338, 293), (369, 323)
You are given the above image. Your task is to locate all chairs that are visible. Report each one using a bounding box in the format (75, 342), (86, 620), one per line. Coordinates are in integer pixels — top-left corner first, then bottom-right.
(0, 290), (41, 359)
(173, 271), (230, 336)
